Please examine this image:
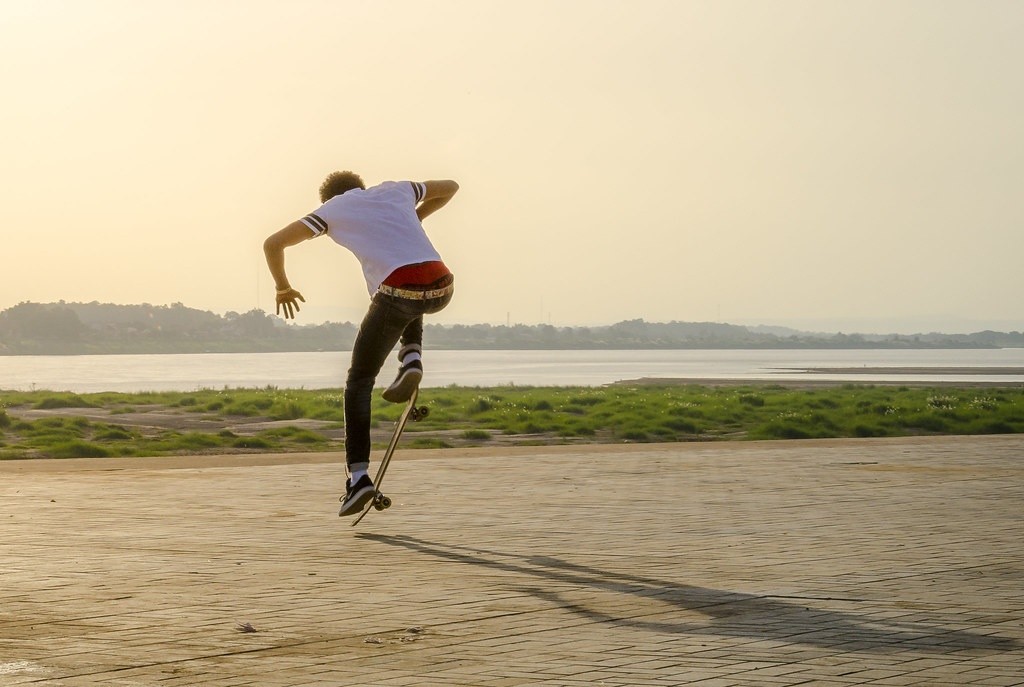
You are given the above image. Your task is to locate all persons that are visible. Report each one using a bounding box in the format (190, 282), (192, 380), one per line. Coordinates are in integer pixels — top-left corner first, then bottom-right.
(263, 171), (460, 517)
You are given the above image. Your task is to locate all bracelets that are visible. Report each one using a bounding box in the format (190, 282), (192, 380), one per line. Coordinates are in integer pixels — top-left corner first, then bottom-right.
(276, 285), (291, 294)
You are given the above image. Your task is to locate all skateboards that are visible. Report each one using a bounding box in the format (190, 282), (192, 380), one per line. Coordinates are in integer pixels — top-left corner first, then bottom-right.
(349, 384), (430, 526)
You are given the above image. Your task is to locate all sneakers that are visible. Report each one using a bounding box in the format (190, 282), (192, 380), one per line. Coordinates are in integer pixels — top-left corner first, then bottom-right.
(381, 359), (423, 404)
(339, 478), (376, 517)
